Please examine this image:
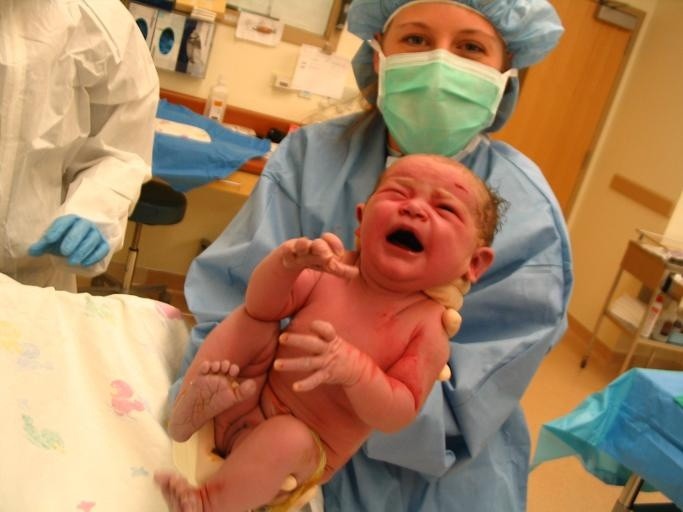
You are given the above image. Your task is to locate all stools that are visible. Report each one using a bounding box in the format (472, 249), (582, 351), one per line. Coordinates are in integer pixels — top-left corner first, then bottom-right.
(77, 180), (188, 302)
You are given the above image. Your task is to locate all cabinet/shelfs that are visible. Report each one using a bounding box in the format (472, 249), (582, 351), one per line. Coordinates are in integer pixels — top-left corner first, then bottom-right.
(580, 226), (683, 376)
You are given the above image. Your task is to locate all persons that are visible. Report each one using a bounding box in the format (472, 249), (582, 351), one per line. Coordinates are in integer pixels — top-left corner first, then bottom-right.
(167, 0), (575, 512)
(153, 150), (510, 512)
(0, 0), (163, 299)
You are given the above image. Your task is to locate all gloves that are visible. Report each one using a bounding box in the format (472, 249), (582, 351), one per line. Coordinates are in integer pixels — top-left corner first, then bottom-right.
(423, 277), (471, 381)
(264, 474), (319, 512)
(27, 215), (109, 268)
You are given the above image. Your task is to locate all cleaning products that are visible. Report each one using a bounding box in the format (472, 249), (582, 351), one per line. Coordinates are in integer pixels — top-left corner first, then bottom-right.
(654, 299), (680, 344)
(640, 294), (666, 338)
(205, 77), (230, 125)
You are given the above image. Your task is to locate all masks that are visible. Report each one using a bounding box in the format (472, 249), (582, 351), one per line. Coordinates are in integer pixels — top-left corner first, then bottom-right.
(367, 38), (518, 156)
